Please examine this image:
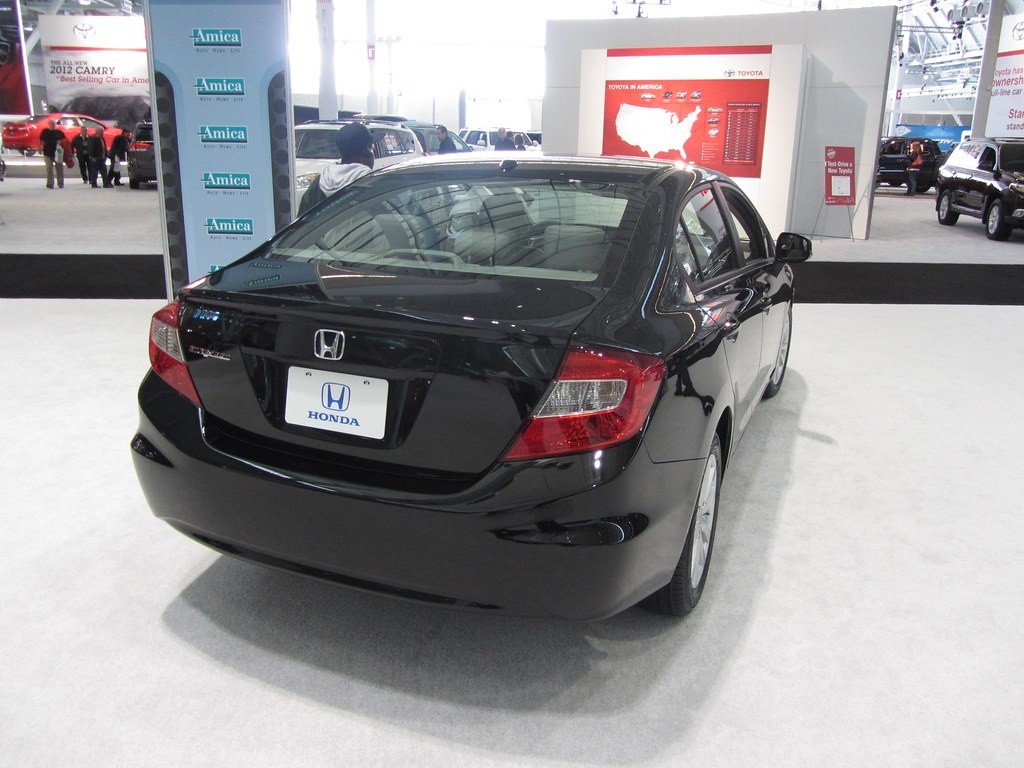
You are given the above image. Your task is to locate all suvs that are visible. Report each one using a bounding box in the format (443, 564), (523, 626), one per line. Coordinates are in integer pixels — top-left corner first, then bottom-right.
(293, 118), (541, 216)
(934, 136), (1024, 241)
(127, 118), (157, 189)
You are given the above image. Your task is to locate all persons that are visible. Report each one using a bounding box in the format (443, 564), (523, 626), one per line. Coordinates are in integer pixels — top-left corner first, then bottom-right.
(495, 127), (515, 150)
(88, 125), (114, 188)
(904, 140), (922, 194)
(297, 122), (377, 218)
(39, 119), (67, 189)
(70, 126), (92, 184)
(507, 130), (515, 142)
(515, 135), (526, 151)
(438, 127), (456, 154)
(946, 144), (956, 154)
(108, 128), (130, 185)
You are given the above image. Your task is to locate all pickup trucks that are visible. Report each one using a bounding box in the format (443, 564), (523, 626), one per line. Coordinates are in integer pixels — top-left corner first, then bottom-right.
(879, 136), (949, 193)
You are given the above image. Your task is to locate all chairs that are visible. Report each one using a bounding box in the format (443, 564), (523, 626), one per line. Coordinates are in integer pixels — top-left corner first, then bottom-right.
(370, 191), (618, 271)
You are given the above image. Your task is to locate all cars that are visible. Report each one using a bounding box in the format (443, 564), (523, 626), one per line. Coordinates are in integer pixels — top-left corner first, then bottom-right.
(640, 93), (656, 99)
(129, 152), (814, 622)
(706, 106), (724, 136)
(663, 91), (702, 100)
(2, 113), (133, 156)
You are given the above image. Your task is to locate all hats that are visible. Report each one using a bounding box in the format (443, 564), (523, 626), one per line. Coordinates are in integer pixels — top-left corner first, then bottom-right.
(337, 122), (385, 155)
